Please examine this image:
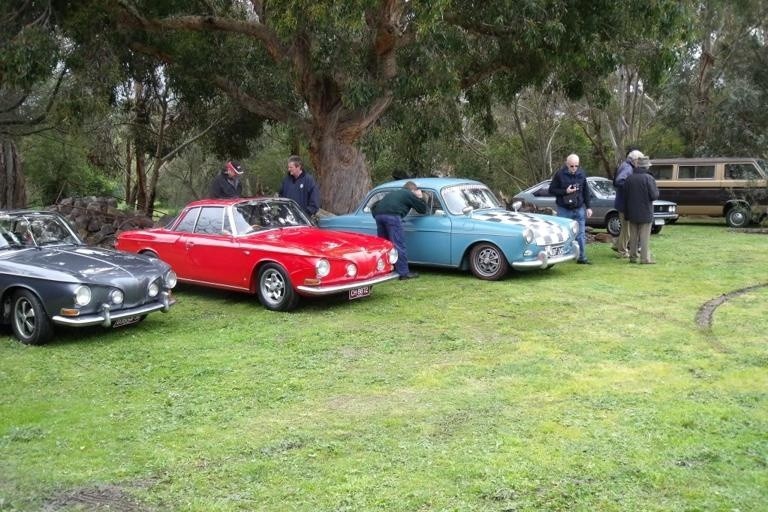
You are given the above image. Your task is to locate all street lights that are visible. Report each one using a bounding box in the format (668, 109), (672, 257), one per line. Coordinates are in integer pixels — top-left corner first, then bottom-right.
(118, 123), (135, 204)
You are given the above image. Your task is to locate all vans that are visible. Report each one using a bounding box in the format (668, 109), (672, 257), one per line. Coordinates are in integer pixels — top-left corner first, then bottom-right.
(648, 158), (767, 227)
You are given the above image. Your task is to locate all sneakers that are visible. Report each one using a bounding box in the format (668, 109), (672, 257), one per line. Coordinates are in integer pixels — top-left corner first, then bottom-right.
(617, 252), (630, 258)
(640, 261), (655, 264)
(630, 257), (637, 263)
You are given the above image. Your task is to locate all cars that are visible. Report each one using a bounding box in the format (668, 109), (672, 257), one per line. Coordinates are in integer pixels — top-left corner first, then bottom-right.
(0, 209), (178, 345)
(113, 197), (400, 311)
(317, 178), (580, 281)
(513, 176), (680, 239)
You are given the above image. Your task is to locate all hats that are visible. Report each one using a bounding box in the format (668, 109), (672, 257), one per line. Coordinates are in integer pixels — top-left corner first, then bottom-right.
(226, 160), (244, 175)
(637, 156), (652, 168)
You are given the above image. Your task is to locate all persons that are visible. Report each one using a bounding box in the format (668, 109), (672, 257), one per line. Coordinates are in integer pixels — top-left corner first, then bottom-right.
(548, 154), (594, 265)
(279, 153), (322, 225)
(210, 161), (244, 198)
(371, 181), (426, 280)
(612, 146), (660, 264)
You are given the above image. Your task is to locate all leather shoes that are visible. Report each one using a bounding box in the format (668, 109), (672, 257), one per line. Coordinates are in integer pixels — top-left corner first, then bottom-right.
(399, 271), (419, 280)
(577, 259), (592, 264)
